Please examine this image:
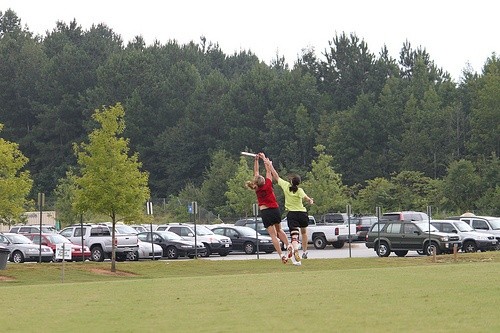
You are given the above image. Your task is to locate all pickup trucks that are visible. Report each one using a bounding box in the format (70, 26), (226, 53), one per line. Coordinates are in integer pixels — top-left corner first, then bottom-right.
(58, 223), (139, 262)
(261, 215), (356, 249)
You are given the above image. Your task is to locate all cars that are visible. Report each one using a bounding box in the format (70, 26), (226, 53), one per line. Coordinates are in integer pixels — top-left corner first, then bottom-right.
(101, 223), (139, 235)
(138, 239), (163, 261)
(0, 232), (54, 264)
(343, 216), (379, 243)
(134, 230), (206, 259)
(211, 226), (277, 254)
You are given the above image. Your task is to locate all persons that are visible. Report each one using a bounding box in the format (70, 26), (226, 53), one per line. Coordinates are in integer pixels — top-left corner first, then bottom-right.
(267, 158), (313, 262)
(247, 152), (293, 264)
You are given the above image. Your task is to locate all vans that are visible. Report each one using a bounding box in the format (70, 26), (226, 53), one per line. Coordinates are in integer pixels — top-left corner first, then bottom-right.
(21, 233), (91, 262)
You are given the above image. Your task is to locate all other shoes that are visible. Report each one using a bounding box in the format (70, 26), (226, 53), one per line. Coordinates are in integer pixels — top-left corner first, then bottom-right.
(302, 252), (308, 258)
(294, 250), (300, 262)
(282, 256), (288, 263)
(286, 245), (293, 258)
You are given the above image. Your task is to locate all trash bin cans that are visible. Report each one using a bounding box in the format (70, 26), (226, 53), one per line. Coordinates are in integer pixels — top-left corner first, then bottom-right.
(0, 247), (11, 270)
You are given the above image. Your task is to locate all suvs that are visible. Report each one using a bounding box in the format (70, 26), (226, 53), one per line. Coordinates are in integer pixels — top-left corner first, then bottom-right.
(365, 220), (463, 257)
(154, 223), (232, 257)
(10, 224), (58, 233)
(446, 216), (500, 250)
(416, 220), (498, 255)
(380, 211), (432, 220)
(234, 219), (291, 248)
